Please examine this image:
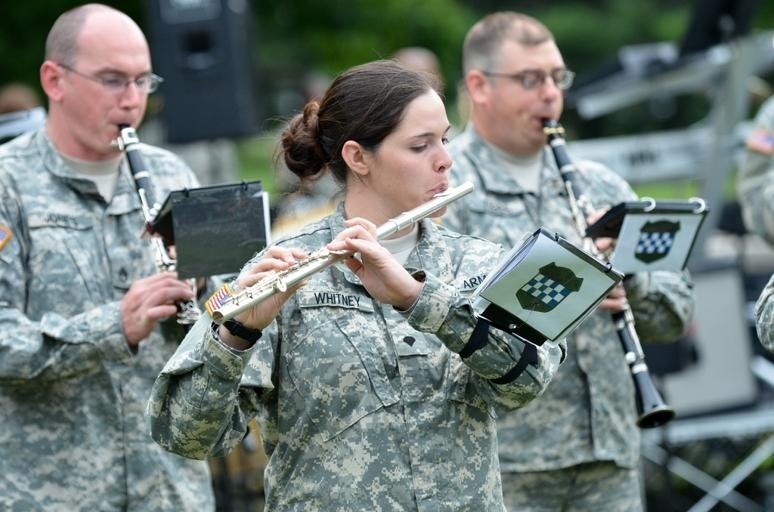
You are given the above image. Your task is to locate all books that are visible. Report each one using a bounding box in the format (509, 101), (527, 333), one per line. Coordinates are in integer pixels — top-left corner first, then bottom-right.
(584, 193), (712, 275)
(470, 226), (625, 352)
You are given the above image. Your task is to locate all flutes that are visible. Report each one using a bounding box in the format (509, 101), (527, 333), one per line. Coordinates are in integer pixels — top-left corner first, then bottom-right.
(211, 180), (475, 325)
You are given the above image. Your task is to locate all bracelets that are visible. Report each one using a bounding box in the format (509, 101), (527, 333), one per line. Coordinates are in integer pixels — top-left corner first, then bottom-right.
(221, 317), (263, 345)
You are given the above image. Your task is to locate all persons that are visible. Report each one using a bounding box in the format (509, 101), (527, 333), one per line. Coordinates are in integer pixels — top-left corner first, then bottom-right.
(140, 56), (567, 511)
(432, 9), (695, 512)
(749, 271), (773, 356)
(736, 89), (771, 246)
(0, 1), (239, 511)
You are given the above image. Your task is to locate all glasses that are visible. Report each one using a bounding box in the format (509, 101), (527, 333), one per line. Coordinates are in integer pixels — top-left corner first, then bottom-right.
(483, 67), (575, 91)
(58, 62), (165, 95)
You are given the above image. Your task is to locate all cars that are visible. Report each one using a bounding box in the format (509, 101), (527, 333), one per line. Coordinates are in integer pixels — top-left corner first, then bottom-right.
(557, 35), (769, 188)
